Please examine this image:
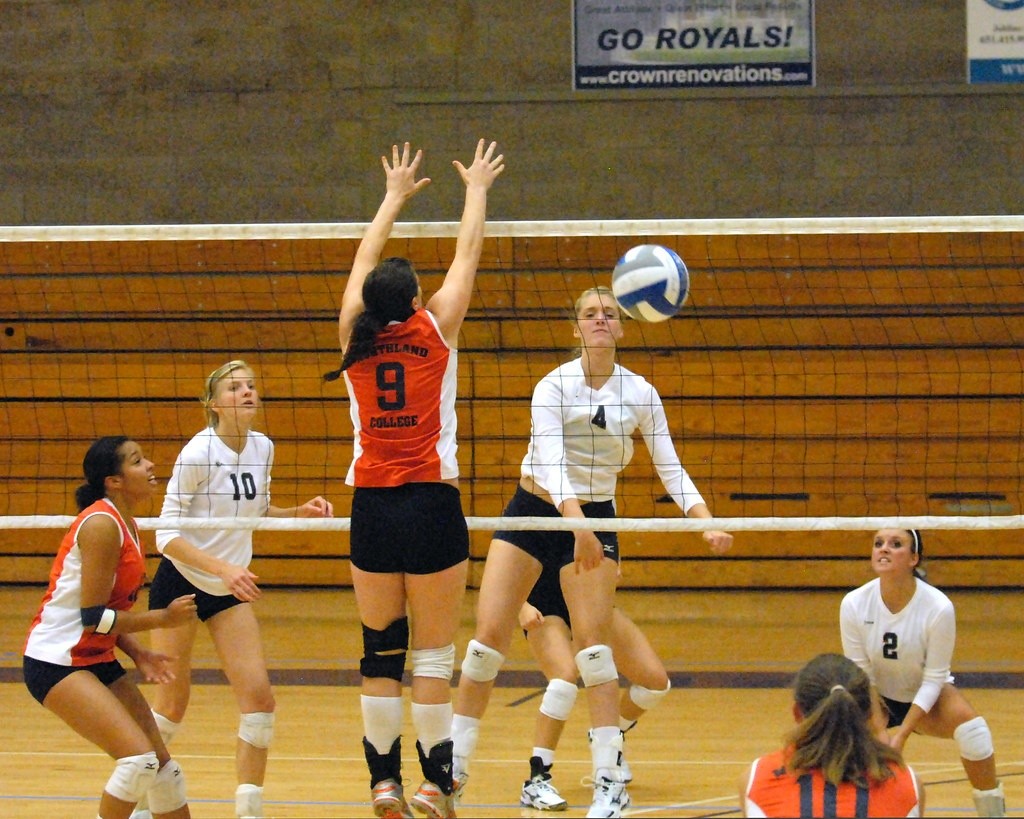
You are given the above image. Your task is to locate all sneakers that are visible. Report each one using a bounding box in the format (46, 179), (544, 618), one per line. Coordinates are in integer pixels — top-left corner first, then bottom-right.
(585, 770), (630, 818)
(411, 780), (457, 819)
(589, 729), (632, 783)
(447, 764), (467, 810)
(521, 779), (566, 811)
(370, 779), (413, 818)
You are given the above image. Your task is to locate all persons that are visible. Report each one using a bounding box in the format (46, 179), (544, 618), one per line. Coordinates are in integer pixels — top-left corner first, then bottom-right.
(840, 530), (1008, 818)
(23, 434), (199, 818)
(128, 359), (334, 818)
(322, 138), (504, 819)
(740, 653), (927, 818)
(450, 283), (733, 818)
(517, 562), (673, 810)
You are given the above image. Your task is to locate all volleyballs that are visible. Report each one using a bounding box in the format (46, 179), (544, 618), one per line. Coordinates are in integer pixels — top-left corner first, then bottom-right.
(610, 244), (690, 323)
